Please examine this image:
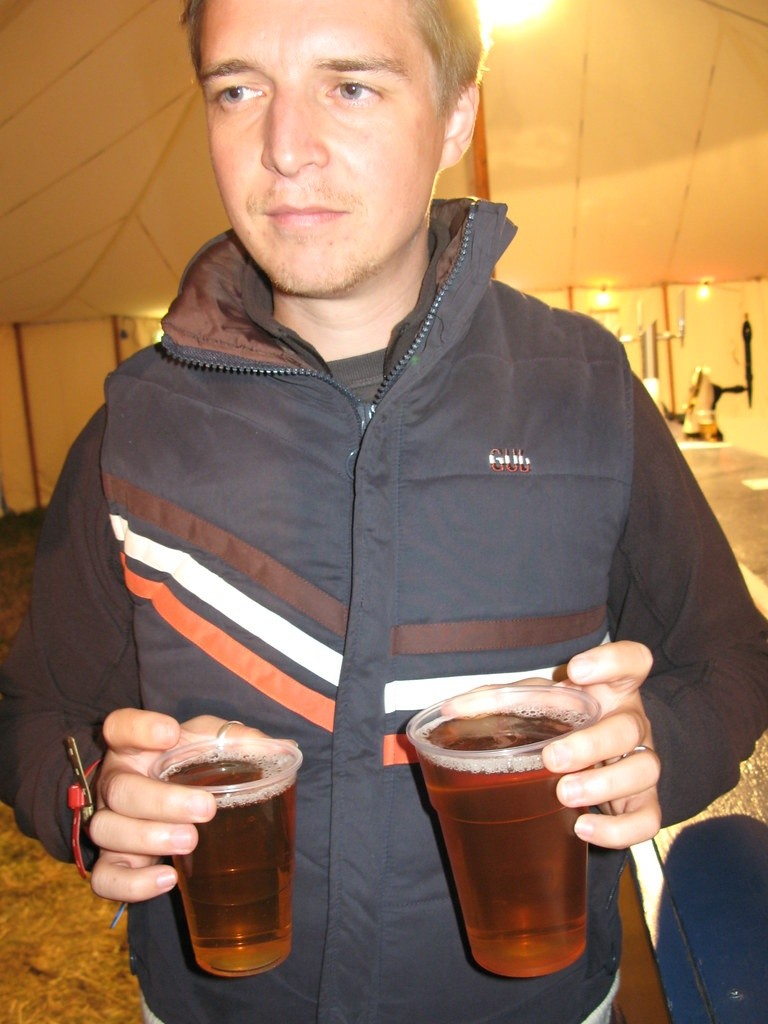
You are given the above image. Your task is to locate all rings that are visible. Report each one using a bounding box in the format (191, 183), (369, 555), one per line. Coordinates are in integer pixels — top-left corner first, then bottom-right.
(621, 745), (651, 759)
(216, 720), (244, 756)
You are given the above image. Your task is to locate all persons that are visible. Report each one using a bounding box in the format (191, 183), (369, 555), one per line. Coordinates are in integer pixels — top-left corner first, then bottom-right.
(0, 0), (768, 1024)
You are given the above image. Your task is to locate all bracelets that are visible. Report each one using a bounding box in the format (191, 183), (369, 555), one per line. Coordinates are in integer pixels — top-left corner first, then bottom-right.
(63, 735), (128, 928)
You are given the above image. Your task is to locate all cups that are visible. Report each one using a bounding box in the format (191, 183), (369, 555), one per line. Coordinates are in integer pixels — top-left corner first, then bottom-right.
(147, 736), (305, 978)
(404, 679), (601, 979)
